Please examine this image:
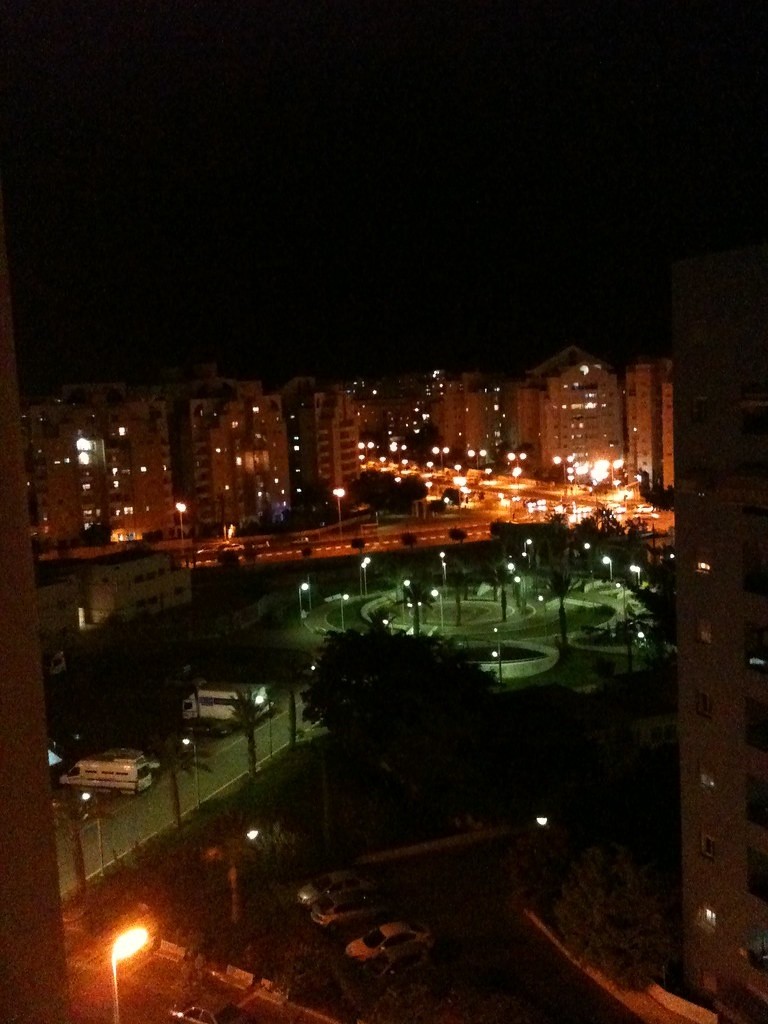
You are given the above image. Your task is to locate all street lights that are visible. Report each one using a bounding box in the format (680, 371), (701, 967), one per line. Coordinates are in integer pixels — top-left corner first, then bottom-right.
(109, 926), (149, 1023)
(294, 437), (659, 686)
(175, 502), (186, 559)
(182, 738), (201, 810)
(81, 792), (106, 877)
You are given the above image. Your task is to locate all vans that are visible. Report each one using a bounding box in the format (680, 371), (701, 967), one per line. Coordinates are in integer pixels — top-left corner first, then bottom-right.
(58, 749), (153, 796)
(184, 684), (268, 723)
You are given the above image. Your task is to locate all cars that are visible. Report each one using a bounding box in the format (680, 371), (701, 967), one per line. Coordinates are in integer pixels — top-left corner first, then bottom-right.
(311, 891), (384, 933)
(346, 922), (435, 964)
(298, 870), (379, 912)
